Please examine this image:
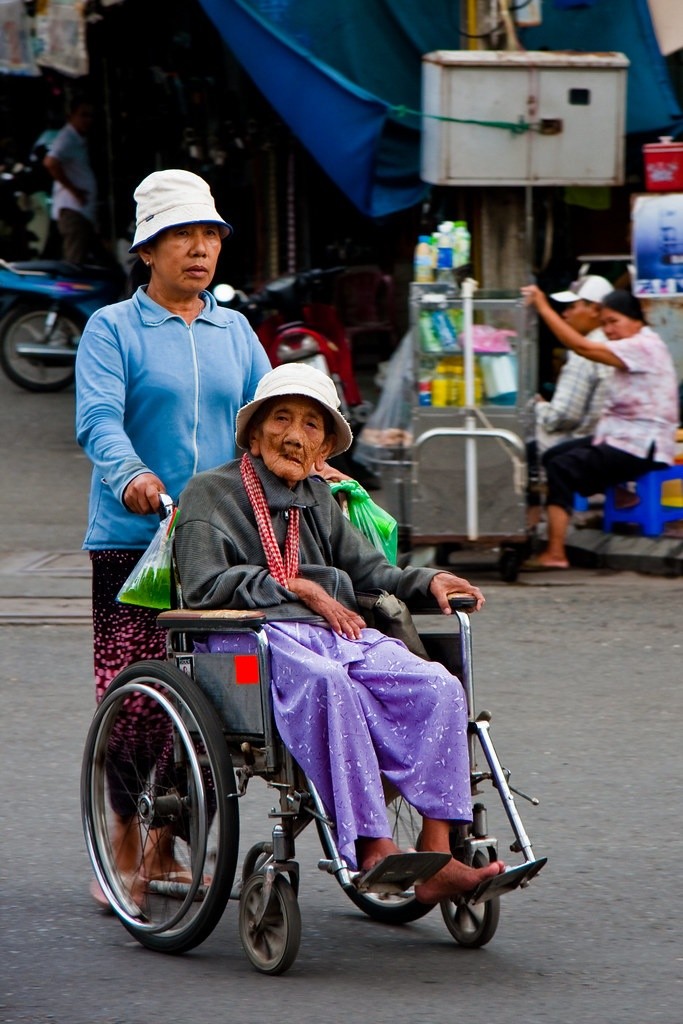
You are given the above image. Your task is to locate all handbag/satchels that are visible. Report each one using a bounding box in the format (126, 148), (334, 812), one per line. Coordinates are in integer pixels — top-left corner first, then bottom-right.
(327, 479), (400, 569)
(358, 326), (417, 467)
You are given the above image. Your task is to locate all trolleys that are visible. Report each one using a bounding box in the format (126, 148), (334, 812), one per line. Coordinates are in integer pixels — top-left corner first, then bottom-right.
(390, 282), (538, 579)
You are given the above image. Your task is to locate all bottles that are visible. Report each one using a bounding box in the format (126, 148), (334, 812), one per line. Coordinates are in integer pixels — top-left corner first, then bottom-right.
(416, 354), (520, 406)
(413, 221), (471, 298)
(421, 303), (468, 352)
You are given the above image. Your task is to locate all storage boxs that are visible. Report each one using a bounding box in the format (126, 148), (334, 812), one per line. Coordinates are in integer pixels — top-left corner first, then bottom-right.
(631, 192), (683, 299)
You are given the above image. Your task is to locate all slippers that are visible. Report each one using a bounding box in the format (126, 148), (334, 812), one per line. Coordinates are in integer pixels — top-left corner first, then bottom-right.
(90, 870), (212, 910)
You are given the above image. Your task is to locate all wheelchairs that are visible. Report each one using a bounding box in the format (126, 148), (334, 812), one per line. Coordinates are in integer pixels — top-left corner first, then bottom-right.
(79, 493), (549, 976)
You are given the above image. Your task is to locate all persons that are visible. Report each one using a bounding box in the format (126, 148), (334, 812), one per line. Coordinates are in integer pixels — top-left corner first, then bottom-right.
(75, 169), (274, 911)
(520, 274), (676, 571)
(43, 94), (97, 264)
(176, 363), (505, 904)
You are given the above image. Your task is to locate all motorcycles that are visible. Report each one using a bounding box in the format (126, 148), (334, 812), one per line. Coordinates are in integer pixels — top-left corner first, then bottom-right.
(212, 261), (372, 490)
(0, 251), (133, 393)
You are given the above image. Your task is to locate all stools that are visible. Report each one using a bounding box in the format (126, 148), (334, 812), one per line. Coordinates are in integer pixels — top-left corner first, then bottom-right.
(575, 483), (628, 512)
(604, 464), (683, 538)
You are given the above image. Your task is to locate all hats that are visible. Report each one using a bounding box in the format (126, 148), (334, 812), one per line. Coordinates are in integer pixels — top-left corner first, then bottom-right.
(549, 275), (616, 304)
(127, 169), (234, 254)
(234, 362), (354, 459)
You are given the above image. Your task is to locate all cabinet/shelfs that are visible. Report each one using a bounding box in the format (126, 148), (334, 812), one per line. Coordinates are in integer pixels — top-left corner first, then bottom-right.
(407, 299), (540, 545)
